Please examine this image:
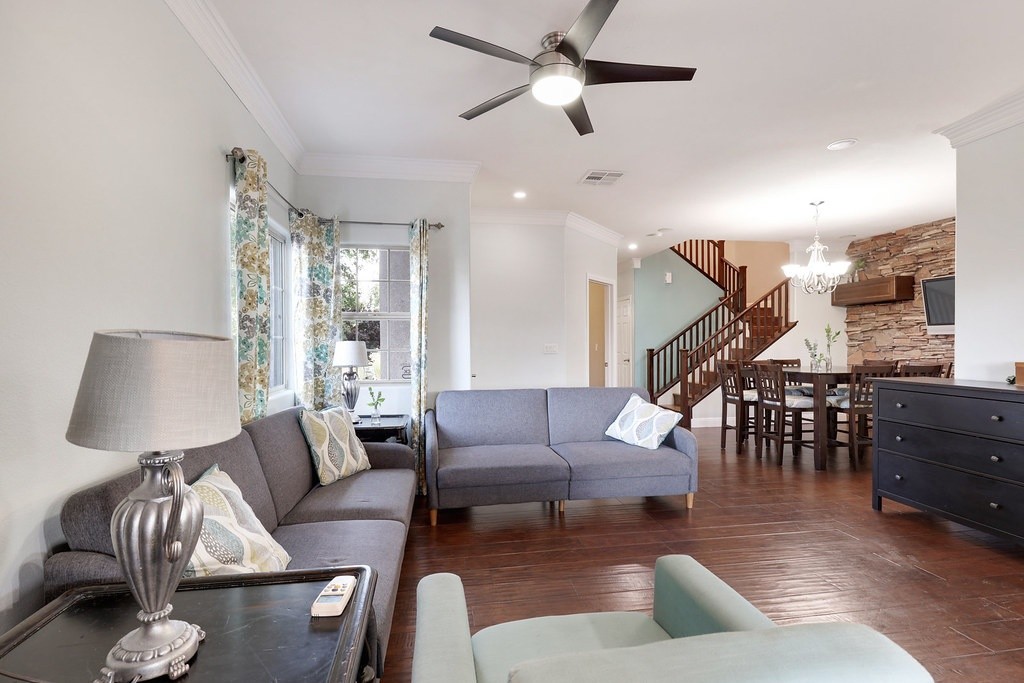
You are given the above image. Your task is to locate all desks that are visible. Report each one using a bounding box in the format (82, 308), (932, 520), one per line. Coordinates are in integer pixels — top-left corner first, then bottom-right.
(0, 565), (378, 683)
(353, 414), (411, 447)
(741, 366), (928, 470)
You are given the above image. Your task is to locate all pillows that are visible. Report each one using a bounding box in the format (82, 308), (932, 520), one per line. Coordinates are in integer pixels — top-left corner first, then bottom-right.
(175, 464), (292, 578)
(604, 393), (685, 451)
(299, 406), (371, 484)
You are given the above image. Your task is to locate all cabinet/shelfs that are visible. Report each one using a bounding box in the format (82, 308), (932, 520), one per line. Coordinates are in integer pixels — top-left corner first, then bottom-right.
(864, 375), (1024, 547)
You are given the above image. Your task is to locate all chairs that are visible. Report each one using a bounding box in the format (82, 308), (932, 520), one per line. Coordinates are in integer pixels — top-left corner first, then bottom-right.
(714, 358), (953, 471)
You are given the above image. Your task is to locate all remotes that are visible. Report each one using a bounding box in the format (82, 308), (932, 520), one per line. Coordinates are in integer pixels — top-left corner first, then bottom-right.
(310, 575), (356, 617)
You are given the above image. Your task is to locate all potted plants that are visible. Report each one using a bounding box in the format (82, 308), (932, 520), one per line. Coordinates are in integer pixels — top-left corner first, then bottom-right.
(822, 326), (841, 369)
(805, 339), (825, 370)
(366, 388), (385, 425)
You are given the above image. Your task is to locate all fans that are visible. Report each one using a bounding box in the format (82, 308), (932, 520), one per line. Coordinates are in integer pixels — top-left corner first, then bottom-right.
(431, 0), (696, 136)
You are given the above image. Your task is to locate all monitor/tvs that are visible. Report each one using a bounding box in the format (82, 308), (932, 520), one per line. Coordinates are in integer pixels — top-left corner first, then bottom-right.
(920, 274), (955, 335)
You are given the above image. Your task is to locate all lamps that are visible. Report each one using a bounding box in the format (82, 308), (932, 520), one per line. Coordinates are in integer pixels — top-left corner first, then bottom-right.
(779, 200), (855, 294)
(332, 339), (372, 423)
(67, 329), (242, 682)
(530, 63), (587, 107)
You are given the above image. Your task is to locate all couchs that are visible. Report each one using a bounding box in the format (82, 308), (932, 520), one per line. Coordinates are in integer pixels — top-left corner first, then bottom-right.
(44, 407), (414, 677)
(412, 554), (936, 683)
(421, 386), (699, 528)
